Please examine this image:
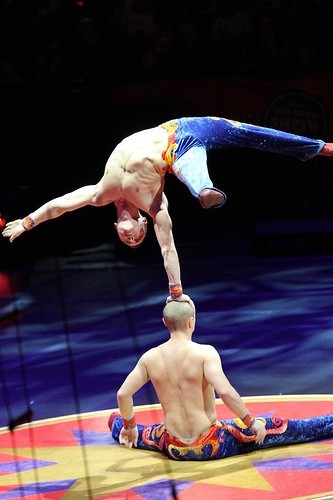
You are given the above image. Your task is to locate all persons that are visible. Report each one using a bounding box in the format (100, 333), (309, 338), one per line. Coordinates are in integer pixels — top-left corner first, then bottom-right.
(2, 115), (333, 308)
(108, 301), (333, 460)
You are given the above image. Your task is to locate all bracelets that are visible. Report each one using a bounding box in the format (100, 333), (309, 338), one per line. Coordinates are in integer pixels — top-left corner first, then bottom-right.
(121, 416), (137, 430)
(169, 280), (183, 297)
(22, 216), (36, 231)
(241, 411), (256, 429)
